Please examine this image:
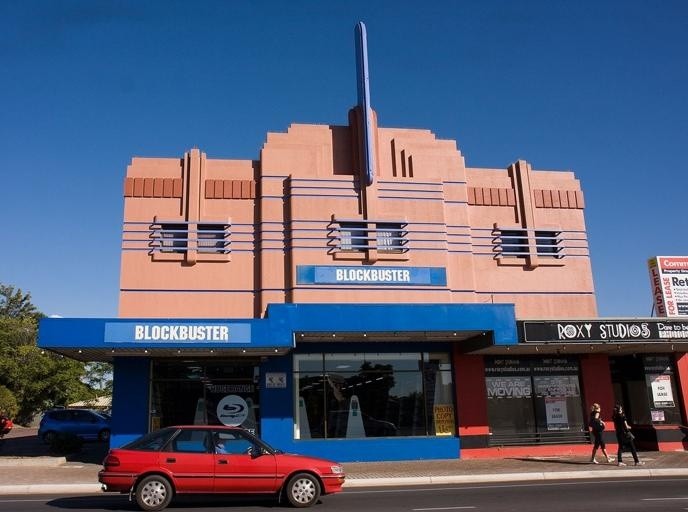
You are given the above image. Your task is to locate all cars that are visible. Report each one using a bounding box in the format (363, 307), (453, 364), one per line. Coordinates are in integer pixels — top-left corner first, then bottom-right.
(37, 408), (111, 449)
(314, 408), (400, 437)
(98, 425), (348, 509)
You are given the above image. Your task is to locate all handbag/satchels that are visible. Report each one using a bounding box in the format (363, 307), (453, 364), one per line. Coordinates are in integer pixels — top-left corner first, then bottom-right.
(624, 432), (636, 442)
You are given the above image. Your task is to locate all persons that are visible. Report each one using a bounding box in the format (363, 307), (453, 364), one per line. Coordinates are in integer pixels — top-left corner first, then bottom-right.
(611, 402), (647, 467)
(588, 402), (616, 465)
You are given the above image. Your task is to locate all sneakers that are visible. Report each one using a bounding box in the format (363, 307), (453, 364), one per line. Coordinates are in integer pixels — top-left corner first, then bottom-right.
(618, 463), (627, 467)
(635, 462), (645, 466)
(592, 460), (599, 464)
(607, 458), (615, 464)
